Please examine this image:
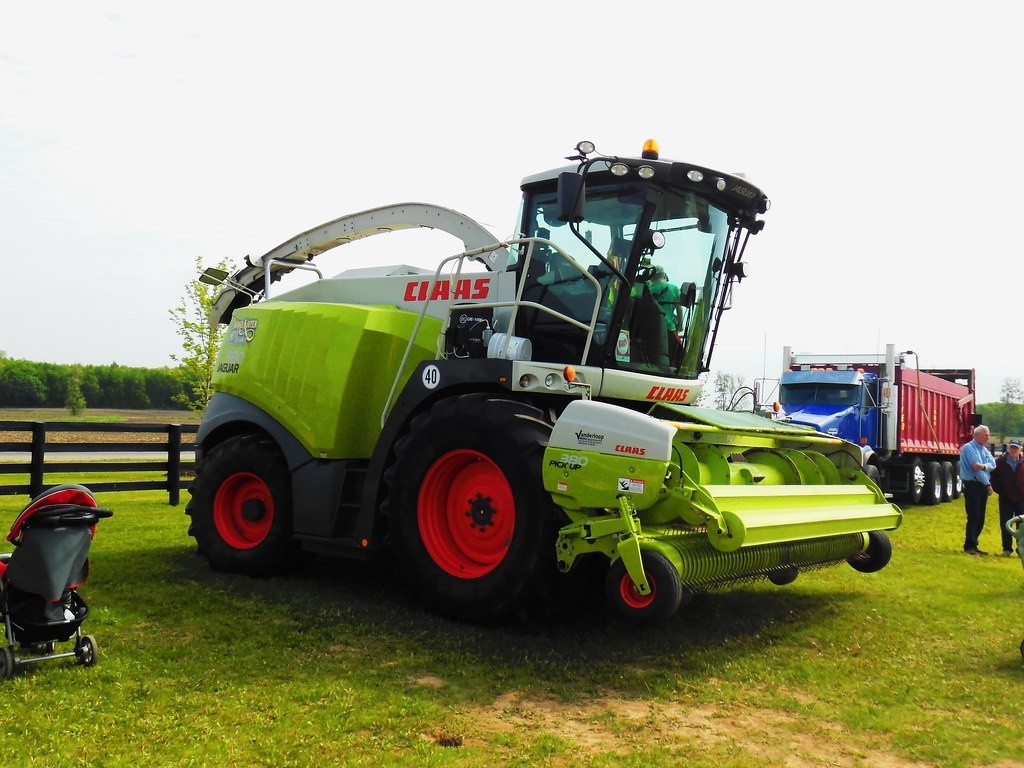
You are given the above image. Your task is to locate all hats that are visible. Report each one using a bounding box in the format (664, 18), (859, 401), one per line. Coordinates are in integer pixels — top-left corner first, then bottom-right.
(648, 266), (670, 282)
(1007, 438), (1023, 449)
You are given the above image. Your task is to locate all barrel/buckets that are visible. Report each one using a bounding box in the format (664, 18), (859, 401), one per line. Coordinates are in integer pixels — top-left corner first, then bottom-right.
(487, 332), (532, 361)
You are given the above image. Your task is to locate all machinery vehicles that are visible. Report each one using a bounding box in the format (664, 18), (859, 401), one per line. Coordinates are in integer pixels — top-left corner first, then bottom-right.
(183, 136), (902, 625)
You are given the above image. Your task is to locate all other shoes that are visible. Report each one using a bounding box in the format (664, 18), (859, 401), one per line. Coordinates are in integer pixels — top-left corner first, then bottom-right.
(975, 547), (989, 555)
(963, 549), (977, 555)
(1002, 551), (1011, 557)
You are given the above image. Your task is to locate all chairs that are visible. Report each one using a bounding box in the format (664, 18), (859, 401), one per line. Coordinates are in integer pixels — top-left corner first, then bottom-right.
(548, 253), (606, 321)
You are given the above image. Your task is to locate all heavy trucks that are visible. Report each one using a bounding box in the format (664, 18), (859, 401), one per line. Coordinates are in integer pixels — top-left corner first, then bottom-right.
(752, 343), (978, 505)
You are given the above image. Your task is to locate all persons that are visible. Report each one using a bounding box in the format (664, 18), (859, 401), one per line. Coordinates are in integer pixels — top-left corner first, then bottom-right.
(959, 424), (997, 555)
(989, 438), (1024, 556)
(647, 265), (685, 346)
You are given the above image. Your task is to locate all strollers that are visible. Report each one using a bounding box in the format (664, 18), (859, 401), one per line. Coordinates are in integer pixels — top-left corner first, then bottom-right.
(0, 483), (115, 677)
